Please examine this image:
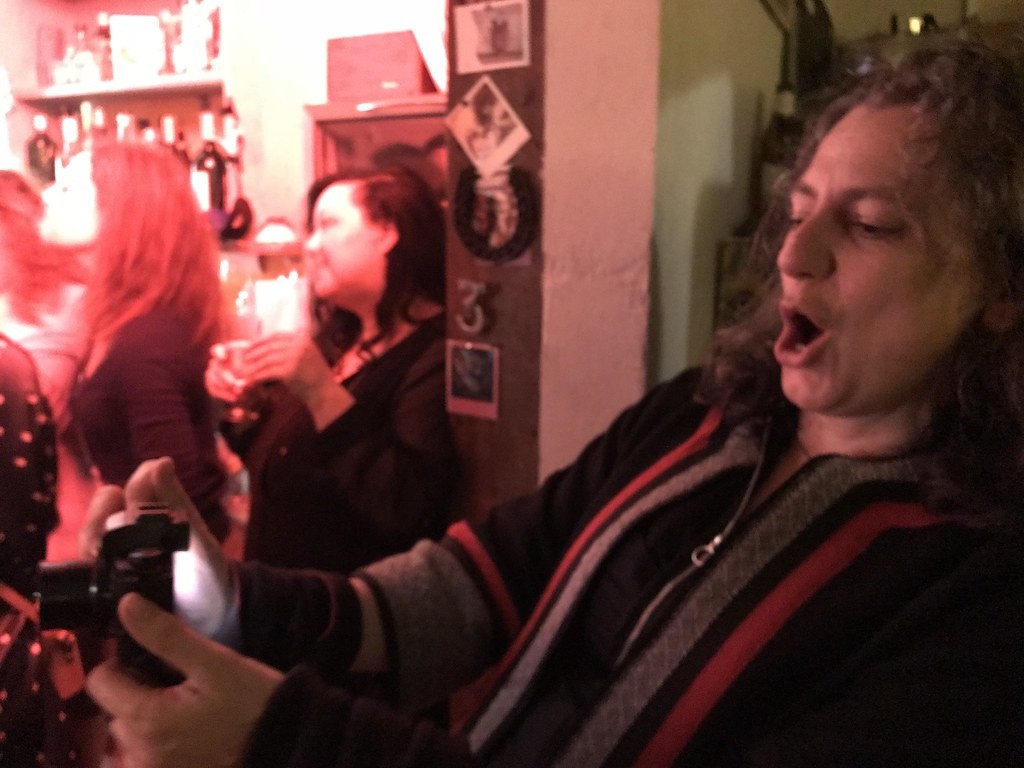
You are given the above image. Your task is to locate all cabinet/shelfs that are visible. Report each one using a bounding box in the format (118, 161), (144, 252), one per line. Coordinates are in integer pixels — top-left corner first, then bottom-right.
(21, 78), (270, 260)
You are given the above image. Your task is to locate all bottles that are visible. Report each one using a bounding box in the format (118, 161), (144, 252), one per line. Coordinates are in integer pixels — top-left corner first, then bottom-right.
(760, 83), (799, 207)
(27, 100), (243, 211)
(64, 1), (211, 83)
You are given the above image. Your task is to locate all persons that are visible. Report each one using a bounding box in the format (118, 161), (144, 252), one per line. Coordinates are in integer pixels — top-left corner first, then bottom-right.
(81, 39), (1024, 767)
(0, 141), (449, 768)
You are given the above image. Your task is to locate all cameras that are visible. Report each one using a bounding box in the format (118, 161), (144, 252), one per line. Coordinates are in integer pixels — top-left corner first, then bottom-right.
(37, 498), (190, 690)
